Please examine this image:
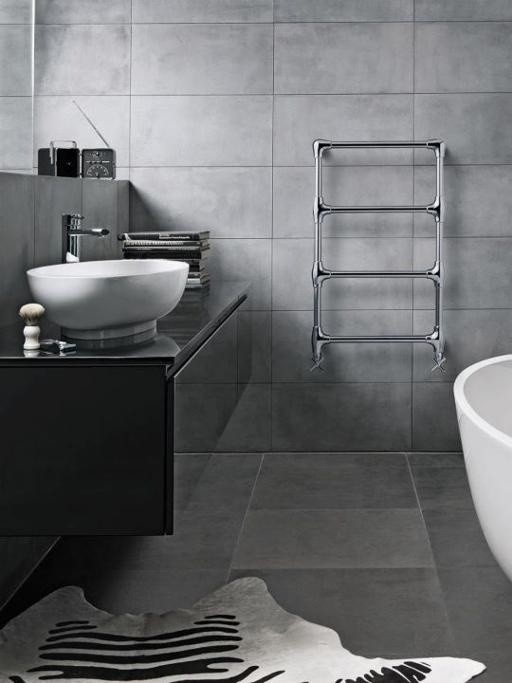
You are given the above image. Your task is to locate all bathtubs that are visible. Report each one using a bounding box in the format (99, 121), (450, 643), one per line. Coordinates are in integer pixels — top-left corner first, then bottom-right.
(454, 354), (512, 578)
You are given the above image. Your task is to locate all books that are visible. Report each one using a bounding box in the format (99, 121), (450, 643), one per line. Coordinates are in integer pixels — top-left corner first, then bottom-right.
(117, 230), (211, 289)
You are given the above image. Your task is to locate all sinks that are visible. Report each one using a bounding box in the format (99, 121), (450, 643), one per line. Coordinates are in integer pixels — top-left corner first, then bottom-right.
(26, 259), (190, 329)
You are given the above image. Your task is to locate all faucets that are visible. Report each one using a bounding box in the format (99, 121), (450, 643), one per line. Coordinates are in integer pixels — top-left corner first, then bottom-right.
(62, 212), (109, 263)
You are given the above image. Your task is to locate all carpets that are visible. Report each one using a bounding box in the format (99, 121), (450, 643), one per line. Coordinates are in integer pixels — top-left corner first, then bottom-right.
(0, 576), (487, 683)
